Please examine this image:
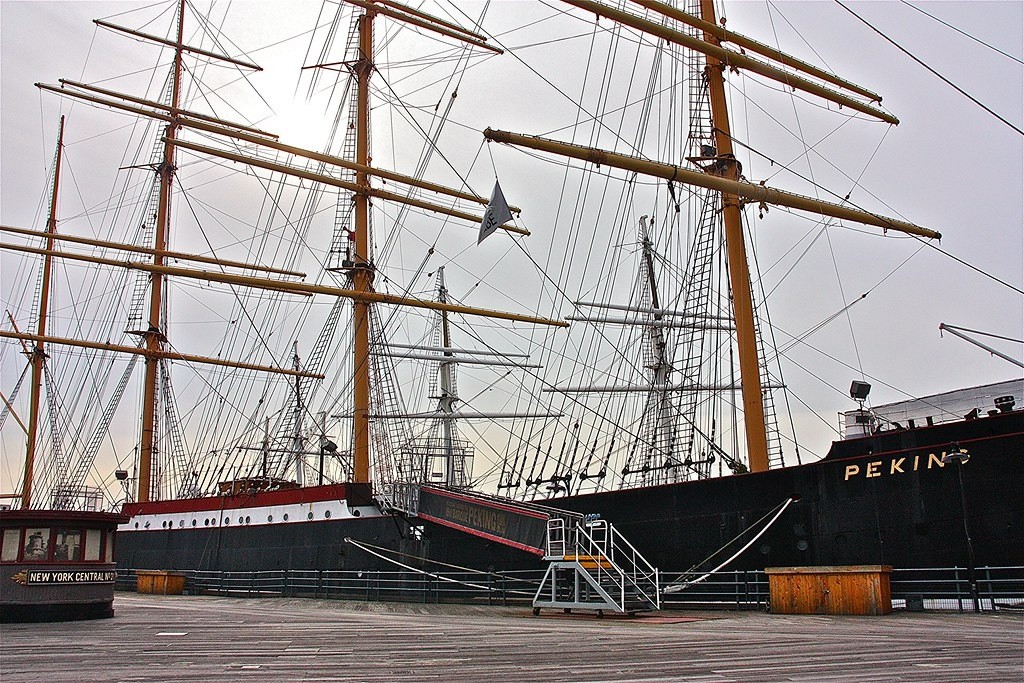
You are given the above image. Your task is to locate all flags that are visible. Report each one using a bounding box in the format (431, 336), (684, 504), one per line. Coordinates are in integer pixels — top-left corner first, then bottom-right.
(478, 180), (513, 246)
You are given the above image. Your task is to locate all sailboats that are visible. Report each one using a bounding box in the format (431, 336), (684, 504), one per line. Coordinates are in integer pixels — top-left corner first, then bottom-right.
(0, 0), (1024, 611)
